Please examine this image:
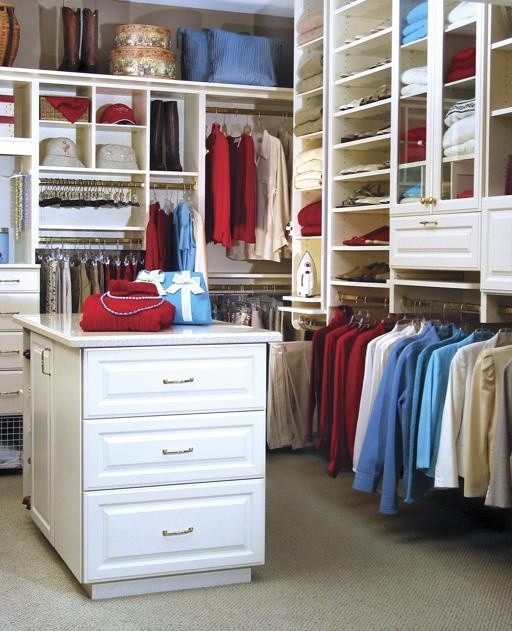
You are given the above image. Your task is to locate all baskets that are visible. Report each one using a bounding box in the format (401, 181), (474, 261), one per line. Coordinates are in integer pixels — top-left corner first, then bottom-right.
(38, 93), (90, 124)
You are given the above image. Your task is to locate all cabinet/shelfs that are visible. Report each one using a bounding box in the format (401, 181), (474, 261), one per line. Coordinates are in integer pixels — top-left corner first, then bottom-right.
(0, 0), (295, 604)
(277, 0), (512, 331)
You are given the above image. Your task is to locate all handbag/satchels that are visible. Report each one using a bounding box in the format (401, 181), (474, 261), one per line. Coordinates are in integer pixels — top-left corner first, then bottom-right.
(136, 269), (214, 328)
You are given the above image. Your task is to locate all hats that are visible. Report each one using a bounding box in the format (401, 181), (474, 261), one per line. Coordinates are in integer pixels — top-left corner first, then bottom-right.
(99, 101), (136, 126)
(96, 143), (140, 172)
(40, 137), (86, 168)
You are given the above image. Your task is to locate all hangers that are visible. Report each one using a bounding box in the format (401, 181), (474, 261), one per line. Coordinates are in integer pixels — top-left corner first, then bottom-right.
(329, 293), (511, 346)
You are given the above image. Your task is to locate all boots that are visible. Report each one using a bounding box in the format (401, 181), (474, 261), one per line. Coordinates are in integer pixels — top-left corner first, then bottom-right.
(56, 6), (81, 73)
(81, 7), (101, 75)
